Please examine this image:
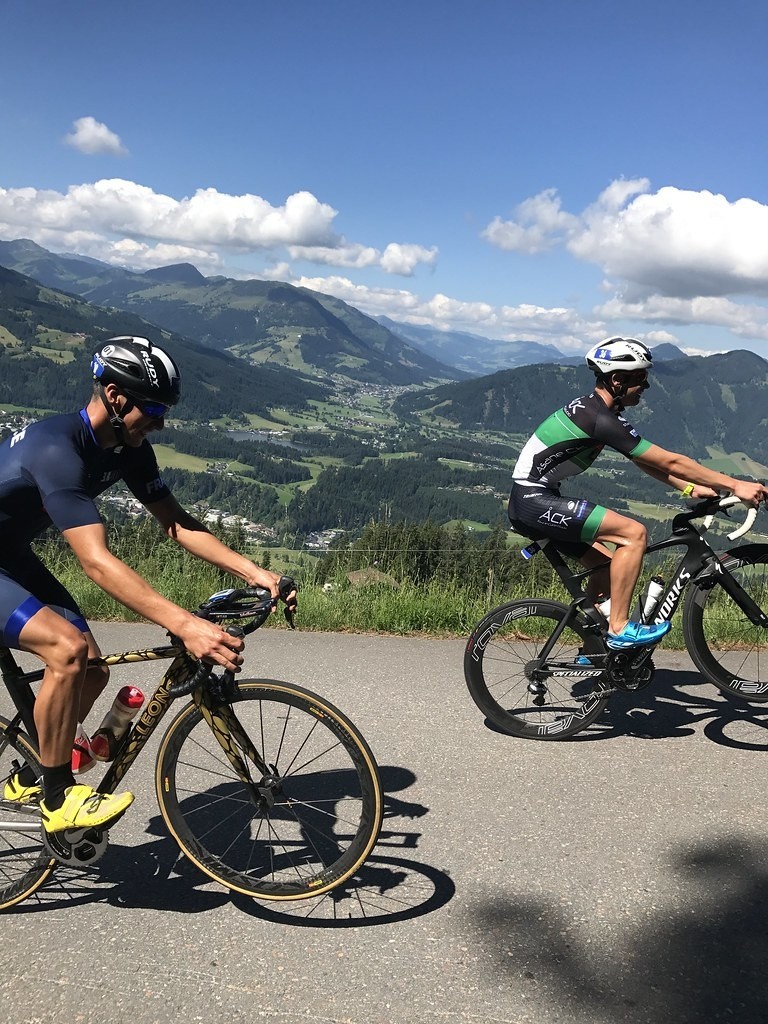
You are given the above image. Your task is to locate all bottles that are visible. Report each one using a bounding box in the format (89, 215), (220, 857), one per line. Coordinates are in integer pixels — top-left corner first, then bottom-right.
(597, 592), (612, 623)
(629, 576), (665, 625)
(71, 722), (97, 775)
(87, 686), (145, 762)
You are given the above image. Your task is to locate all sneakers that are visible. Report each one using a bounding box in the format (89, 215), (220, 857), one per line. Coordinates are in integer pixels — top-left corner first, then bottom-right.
(575, 655), (592, 665)
(606, 620), (671, 651)
(40, 784), (135, 832)
(3, 773), (43, 804)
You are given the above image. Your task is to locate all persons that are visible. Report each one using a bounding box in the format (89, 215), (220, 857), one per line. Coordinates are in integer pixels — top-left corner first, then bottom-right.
(0, 336), (297, 833)
(508, 336), (768, 669)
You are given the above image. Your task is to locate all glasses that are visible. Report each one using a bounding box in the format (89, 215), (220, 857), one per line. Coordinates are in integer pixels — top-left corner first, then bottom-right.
(120, 389), (170, 420)
(624, 371), (649, 384)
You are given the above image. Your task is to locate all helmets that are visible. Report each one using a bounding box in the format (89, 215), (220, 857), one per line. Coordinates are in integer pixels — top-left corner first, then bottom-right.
(90, 335), (182, 409)
(585, 335), (654, 374)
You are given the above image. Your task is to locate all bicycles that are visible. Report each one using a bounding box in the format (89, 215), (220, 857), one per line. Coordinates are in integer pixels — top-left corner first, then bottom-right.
(0, 574), (387, 912)
(463, 483), (768, 740)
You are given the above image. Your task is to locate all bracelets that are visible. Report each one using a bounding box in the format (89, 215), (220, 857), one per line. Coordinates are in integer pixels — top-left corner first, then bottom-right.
(681, 481), (695, 497)
